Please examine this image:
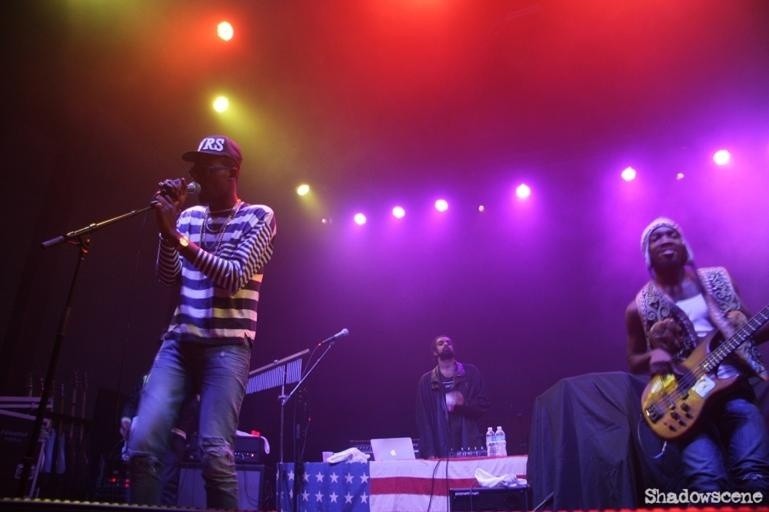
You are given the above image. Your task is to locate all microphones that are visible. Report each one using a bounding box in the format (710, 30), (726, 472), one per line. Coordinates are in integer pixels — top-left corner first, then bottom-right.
(319, 326), (350, 345)
(184, 180), (201, 195)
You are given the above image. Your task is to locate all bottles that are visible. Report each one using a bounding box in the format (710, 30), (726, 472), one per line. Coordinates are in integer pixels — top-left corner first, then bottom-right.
(485, 426), (508, 458)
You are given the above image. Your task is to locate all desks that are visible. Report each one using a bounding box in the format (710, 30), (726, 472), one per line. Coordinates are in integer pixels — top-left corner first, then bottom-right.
(272, 454), (531, 512)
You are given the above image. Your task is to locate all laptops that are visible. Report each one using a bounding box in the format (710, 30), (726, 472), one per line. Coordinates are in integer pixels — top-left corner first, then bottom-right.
(369, 437), (417, 461)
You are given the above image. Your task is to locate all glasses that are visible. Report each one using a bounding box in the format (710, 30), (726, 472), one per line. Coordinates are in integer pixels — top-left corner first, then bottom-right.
(189, 162), (229, 178)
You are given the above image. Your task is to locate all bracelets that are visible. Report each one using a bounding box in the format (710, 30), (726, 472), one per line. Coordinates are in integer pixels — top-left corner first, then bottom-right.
(160, 231), (168, 239)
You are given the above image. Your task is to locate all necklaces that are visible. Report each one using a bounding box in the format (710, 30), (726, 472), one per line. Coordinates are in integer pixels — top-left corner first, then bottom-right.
(199, 199), (244, 261)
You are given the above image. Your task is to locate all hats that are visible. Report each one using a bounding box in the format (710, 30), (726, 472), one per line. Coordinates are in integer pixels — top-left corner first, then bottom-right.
(182, 135), (243, 169)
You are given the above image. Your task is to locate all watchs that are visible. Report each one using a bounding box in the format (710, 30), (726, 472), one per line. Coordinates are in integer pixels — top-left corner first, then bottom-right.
(175, 237), (189, 251)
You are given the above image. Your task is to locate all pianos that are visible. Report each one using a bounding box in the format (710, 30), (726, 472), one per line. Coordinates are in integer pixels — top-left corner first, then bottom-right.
(0, 396), (54, 501)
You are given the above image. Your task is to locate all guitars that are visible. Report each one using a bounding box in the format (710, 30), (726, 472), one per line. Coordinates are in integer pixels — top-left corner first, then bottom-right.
(640, 303), (767, 439)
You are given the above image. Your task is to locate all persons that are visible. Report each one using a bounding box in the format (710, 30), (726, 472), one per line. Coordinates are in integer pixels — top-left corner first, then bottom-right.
(416, 333), (491, 459)
(127, 134), (276, 510)
(120, 372), (194, 459)
(623, 217), (769, 507)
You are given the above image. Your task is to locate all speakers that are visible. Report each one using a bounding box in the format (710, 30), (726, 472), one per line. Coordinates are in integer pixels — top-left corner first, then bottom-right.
(449, 483), (534, 511)
